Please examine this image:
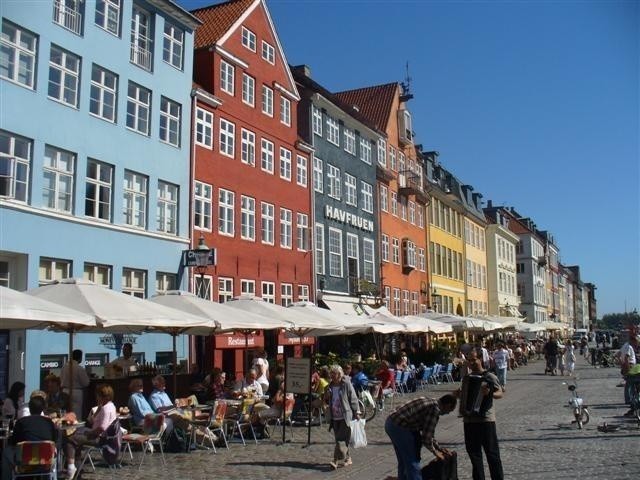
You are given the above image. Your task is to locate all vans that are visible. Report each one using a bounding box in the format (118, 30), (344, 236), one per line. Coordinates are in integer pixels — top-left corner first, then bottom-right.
(572, 328), (589, 343)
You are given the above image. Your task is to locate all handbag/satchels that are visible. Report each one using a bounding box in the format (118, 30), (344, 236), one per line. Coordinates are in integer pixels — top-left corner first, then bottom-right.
(350, 419), (367, 449)
(323, 402), (331, 424)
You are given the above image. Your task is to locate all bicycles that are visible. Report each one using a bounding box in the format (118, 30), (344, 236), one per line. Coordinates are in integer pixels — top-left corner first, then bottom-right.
(622, 374), (640, 423)
(562, 374), (590, 430)
(586, 349), (621, 369)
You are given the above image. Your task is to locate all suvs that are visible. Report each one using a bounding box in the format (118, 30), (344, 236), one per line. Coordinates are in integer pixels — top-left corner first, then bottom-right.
(595, 331), (612, 344)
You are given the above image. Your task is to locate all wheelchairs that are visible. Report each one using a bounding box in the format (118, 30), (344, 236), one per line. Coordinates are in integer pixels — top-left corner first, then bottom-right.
(354, 379), (384, 420)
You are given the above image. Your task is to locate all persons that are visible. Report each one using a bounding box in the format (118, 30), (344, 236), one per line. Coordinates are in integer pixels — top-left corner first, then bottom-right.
(1, 342), (504, 480)
(471, 326), (640, 405)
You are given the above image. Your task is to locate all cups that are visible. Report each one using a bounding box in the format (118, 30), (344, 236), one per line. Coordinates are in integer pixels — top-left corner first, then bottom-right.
(2, 419), (10, 430)
(60, 410), (66, 419)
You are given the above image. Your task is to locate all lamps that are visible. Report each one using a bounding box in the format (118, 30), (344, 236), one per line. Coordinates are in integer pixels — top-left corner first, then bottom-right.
(420, 280), (427, 297)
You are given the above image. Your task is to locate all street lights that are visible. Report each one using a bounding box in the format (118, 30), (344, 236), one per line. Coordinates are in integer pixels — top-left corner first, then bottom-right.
(431, 287), (440, 351)
(505, 302), (510, 338)
(194, 230), (211, 378)
(549, 314), (556, 335)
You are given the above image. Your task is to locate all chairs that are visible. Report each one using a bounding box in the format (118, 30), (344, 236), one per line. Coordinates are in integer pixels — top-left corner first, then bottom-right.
(293, 362), (455, 428)
(1, 374), (295, 477)
